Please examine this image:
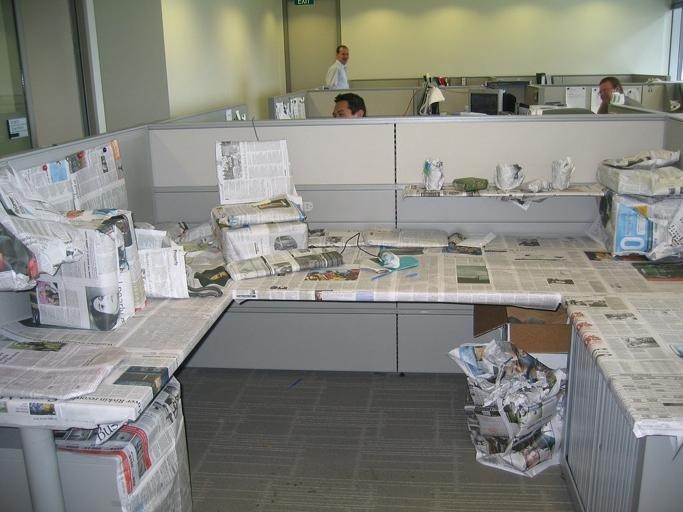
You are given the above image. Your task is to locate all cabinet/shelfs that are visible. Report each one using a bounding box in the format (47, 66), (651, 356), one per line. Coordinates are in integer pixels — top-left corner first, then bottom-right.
(188, 300), (397, 373)
(397, 304), (570, 378)
(561, 299), (682, 512)
(0, 376), (192, 512)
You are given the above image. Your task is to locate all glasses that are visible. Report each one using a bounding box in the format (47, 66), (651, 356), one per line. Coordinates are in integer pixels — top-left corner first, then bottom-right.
(598, 87), (614, 96)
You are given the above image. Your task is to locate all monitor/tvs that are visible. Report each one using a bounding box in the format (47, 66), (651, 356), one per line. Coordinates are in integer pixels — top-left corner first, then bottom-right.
(466, 89), (516, 115)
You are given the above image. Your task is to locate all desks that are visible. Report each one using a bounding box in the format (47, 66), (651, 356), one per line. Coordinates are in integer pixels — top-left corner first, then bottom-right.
(232, 220), (678, 306)
(1, 219), (235, 510)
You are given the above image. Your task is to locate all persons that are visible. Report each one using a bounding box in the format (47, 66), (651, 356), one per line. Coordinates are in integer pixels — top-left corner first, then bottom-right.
(332, 93), (366, 118)
(325, 45), (351, 90)
(596, 77), (644, 113)
